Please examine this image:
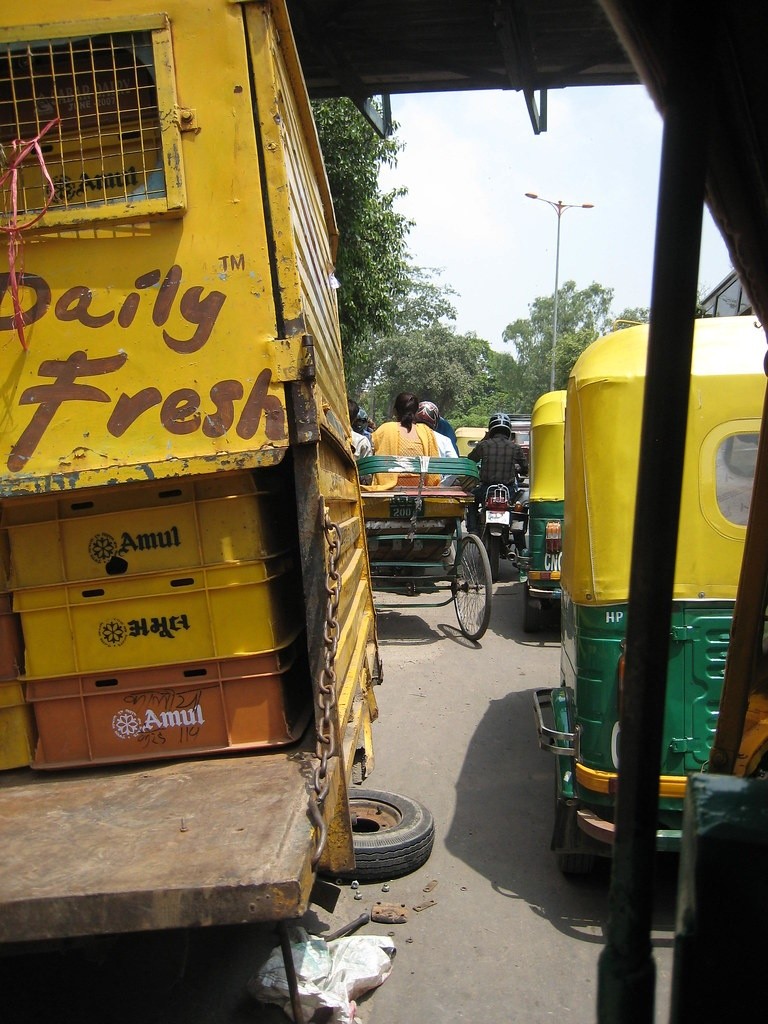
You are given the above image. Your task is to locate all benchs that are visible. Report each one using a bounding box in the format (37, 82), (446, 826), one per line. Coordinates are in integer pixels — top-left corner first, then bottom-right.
(359, 485), (476, 519)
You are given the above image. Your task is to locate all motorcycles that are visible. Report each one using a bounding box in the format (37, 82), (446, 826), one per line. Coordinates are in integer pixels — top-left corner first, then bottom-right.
(463, 438), (529, 586)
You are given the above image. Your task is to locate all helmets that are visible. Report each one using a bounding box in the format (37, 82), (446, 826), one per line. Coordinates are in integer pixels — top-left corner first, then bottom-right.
(489, 413), (512, 433)
(413, 401), (440, 430)
(352, 408), (368, 433)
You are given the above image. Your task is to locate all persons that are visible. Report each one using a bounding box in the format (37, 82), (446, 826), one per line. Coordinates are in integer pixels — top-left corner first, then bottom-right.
(466, 412), (529, 556)
(347, 390), (461, 487)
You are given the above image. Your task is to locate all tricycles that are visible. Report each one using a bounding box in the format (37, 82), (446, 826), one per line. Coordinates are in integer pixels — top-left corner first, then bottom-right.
(355, 454), (494, 642)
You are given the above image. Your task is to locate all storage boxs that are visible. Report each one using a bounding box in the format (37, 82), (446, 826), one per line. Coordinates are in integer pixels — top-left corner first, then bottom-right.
(1, 118), (317, 770)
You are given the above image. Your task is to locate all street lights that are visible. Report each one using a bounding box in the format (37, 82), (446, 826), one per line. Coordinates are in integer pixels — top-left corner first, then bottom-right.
(525, 192), (594, 392)
